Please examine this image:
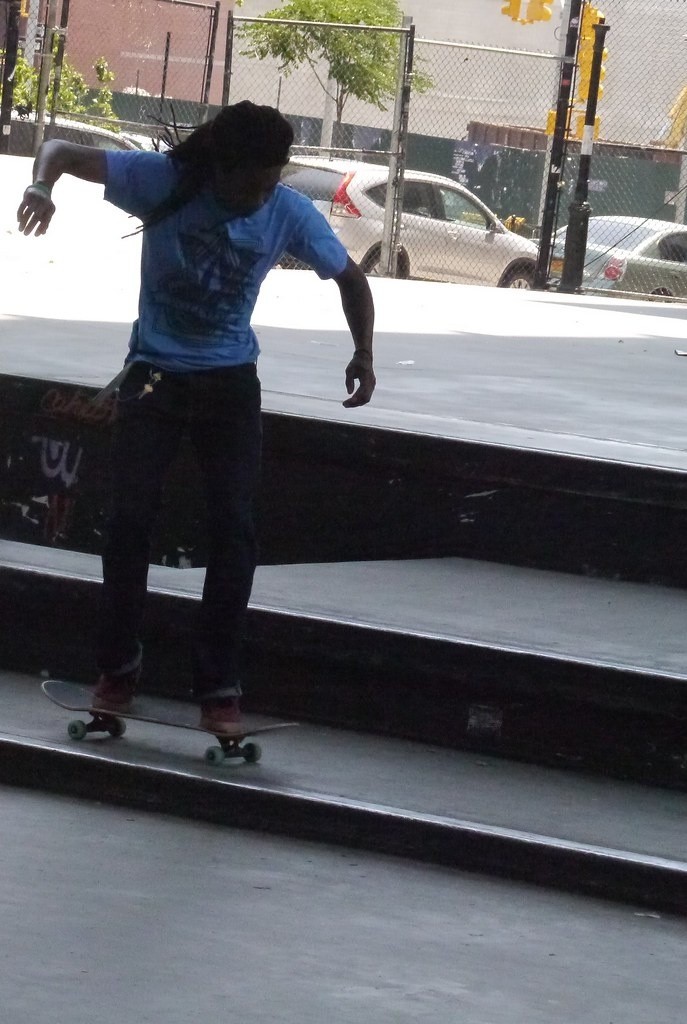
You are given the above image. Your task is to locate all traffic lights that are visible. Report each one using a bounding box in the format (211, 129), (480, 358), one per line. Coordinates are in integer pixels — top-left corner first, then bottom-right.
(577, 6), (609, 106)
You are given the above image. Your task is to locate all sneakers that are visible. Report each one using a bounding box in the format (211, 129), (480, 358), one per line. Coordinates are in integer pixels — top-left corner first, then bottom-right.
(199, 696), (242, 735)
(93, 661), (142, 712)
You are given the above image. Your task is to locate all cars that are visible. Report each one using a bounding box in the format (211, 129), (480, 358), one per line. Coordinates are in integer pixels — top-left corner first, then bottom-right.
(526, 215), (687, 300)
(0, 109), (179, 191)
(277, 156), (548, 291)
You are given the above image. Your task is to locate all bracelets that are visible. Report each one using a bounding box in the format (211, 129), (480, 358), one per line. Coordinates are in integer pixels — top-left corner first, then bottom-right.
(27, 183), (51, 198)
(354, 348), (373, 361)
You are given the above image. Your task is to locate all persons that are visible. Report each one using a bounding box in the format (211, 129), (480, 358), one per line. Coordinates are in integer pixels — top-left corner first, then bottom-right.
(18, 100), (375, 733)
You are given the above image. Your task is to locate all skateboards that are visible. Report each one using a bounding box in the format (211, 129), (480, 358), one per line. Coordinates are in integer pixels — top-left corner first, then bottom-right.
(37, 675), (306, 764)
